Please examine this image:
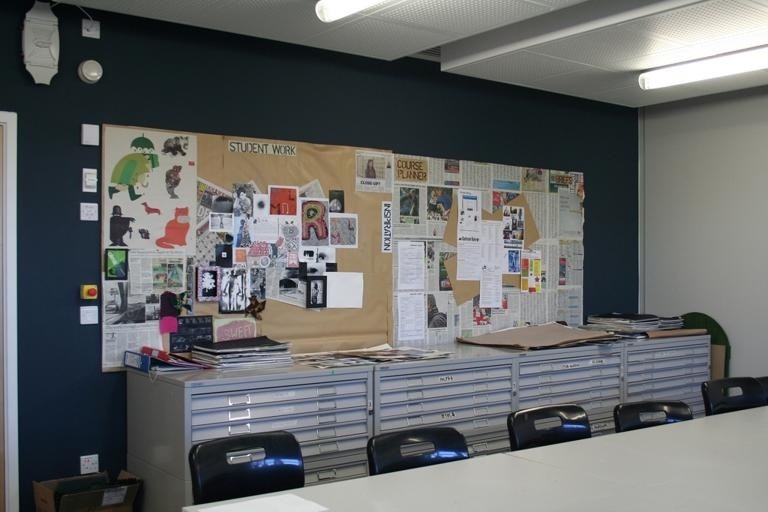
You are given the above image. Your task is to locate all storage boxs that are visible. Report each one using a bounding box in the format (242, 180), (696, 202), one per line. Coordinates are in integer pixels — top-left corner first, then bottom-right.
(31, 468), (144, 511)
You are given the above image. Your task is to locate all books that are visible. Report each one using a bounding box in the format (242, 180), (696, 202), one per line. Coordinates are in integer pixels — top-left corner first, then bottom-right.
(191, 336), (292, 369)
(587, 314), (685, 332)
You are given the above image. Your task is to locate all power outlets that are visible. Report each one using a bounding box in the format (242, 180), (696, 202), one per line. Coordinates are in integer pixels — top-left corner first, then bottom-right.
(82, 19), (100, 39)
(80, 453), (100, 475)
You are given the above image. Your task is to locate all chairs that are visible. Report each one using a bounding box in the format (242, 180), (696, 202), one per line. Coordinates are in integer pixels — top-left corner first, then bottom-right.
(188, 427), (305, 503)
(365, 424), (472, 475)
(698, 373), (762, 414)
(613, 396), (693, 433)
(502, 403), (592, 450)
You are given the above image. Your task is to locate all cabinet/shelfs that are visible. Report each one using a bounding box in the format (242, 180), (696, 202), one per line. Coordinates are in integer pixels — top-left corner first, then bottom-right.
(512, 338), (630, 442)
(626, 329), (710, 415)
(377, 358), (518, 480)
(127, 359), (372, 512)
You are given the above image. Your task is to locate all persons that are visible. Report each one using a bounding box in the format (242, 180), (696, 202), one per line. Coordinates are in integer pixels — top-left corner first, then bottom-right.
(366, 159), (375, 178)
(224, 270), (240, 311)
(233, 187), (251, 219)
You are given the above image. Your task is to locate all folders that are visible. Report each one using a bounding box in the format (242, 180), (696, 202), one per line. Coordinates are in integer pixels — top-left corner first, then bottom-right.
(124, 346), (212, 374)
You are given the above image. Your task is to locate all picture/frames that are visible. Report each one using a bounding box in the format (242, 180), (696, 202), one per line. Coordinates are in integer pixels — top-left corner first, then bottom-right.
(196, 265), (220, 302)
(305, 276), (328, 308)
(105, 247), (128, 281)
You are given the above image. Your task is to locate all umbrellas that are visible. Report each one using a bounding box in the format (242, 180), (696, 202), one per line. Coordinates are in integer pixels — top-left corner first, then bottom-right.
(130, 134), (154, 187)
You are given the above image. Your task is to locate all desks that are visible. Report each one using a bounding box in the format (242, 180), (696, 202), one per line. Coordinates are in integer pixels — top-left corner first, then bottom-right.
(182, 403), (766, 512)
